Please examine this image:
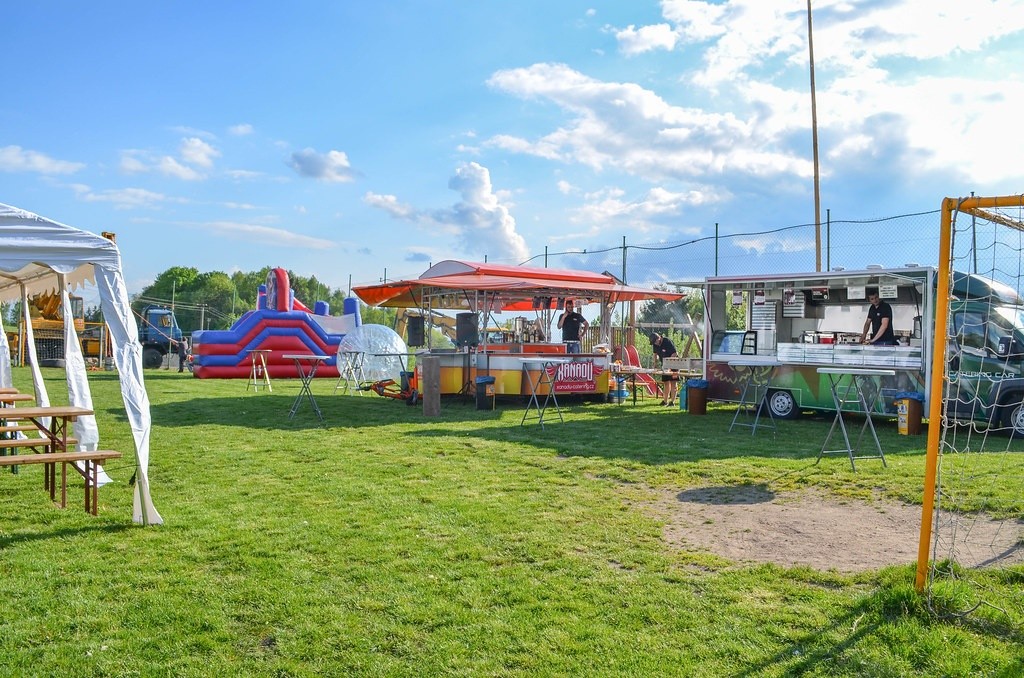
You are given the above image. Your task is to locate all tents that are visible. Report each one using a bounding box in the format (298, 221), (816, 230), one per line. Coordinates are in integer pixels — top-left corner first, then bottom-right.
(0, 202), (163, 528)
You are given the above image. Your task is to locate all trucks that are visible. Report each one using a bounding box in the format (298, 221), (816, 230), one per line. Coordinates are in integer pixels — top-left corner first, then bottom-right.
(668, 264), (1024, 439)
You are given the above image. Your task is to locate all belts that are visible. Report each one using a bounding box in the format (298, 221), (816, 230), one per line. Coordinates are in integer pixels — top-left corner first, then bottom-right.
(563, 339), (580, 343)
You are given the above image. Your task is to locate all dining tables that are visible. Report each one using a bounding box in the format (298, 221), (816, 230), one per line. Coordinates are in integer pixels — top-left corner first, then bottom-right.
(246, 350), (273, 393)
(336, 351), (367, 397)
(283, 354), (332, 422)
(519, 357), (574, 431)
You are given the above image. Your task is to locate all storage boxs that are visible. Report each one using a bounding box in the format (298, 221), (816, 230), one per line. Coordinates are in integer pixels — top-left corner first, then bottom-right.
(897, 398), (922, 436)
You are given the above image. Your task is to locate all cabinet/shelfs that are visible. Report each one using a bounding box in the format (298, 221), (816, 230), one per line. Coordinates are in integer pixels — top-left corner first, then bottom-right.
(711, 330), (757, 355)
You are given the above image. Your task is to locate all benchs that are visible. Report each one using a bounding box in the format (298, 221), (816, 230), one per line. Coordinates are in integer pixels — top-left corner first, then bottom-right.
(0, 417), (120, 516)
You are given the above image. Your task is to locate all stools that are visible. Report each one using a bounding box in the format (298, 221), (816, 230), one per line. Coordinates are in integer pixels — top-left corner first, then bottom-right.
(655, 381), (676, 399)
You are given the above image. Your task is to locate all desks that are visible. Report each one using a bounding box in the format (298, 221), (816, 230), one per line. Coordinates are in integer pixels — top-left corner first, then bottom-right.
(615, 369), (703, 413)
(816, 367), (896, 472)
(0, 388), (94, 509)
(728, 361), (784, 437)
(625, 382), (649, 403)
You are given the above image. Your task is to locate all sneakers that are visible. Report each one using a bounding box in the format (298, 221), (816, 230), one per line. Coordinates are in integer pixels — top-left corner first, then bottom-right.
(668, 403), (674, 406)
(659, 400), (667, 406)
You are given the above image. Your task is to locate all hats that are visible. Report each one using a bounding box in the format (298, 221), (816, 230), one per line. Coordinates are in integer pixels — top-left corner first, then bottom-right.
(649, 333), (660, 345)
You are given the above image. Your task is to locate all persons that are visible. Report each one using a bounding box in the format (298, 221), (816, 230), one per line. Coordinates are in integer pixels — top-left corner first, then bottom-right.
(557, 300), (590, 353)
(649, 333), (681, 407)
(168, 336), (188, 371)
(860, 290), (894, 346)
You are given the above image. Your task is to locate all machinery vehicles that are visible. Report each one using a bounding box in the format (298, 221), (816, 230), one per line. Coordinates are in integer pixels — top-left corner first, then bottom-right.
(395, 307), (516, 356)
(5, 231), (116, 367)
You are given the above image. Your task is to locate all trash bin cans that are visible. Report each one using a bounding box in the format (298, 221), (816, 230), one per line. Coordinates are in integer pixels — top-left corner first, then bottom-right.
(475, 375), (496, 411)
(687, 378), (710, 415)
(895, 391), (926, 435)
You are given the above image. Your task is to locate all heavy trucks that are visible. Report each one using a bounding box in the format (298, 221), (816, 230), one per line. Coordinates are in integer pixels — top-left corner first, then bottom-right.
(136, 305), (184, 370)
